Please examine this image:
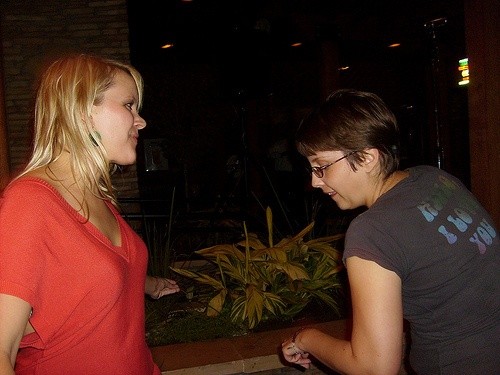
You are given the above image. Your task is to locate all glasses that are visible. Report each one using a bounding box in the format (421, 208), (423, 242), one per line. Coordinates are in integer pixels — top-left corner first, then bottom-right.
(306, 151), (357, 178)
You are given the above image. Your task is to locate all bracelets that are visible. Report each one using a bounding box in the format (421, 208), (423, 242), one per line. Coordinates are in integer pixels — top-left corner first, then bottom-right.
(291, 326), (314, 355)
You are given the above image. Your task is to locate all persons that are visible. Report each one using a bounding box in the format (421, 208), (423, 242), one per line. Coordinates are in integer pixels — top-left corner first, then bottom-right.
(279, 90), (500, 375)
(0, 50), (164, 375)
